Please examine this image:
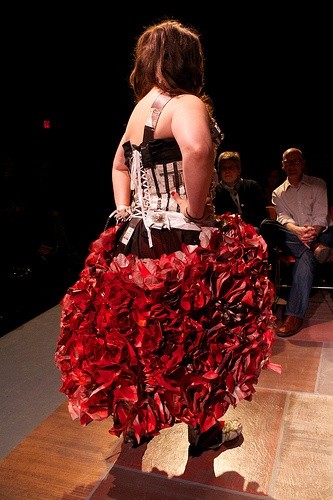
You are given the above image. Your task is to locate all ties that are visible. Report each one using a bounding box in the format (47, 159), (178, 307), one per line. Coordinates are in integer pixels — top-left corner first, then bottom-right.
(229, 189), (238, 207)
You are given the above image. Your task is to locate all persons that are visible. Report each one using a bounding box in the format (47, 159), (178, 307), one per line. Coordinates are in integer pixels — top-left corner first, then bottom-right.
(259, 148), (333, 337)
(213, 151), (267, 227)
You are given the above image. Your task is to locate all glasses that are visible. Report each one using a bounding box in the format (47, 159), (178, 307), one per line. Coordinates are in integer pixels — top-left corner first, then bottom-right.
(221, 166), (235, 171)
(284, 158), (305, 163)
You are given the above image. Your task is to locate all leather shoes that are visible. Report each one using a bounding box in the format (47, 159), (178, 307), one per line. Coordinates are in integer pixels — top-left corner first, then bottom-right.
(315, 245), (333, 263)
(277, 316), (303, 336)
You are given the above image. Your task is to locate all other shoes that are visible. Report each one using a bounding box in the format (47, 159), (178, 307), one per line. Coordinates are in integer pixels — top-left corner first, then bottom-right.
(126, 429), (134, 442)
(188, 419), (241, 446)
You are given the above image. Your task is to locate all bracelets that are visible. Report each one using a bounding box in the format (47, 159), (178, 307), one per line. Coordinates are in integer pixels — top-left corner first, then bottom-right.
(186, 207), (205, 220)
(54, 19), (275, 449)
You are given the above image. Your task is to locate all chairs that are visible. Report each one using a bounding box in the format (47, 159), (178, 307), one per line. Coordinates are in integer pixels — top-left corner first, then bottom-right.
(266, 205), (333, 296)
